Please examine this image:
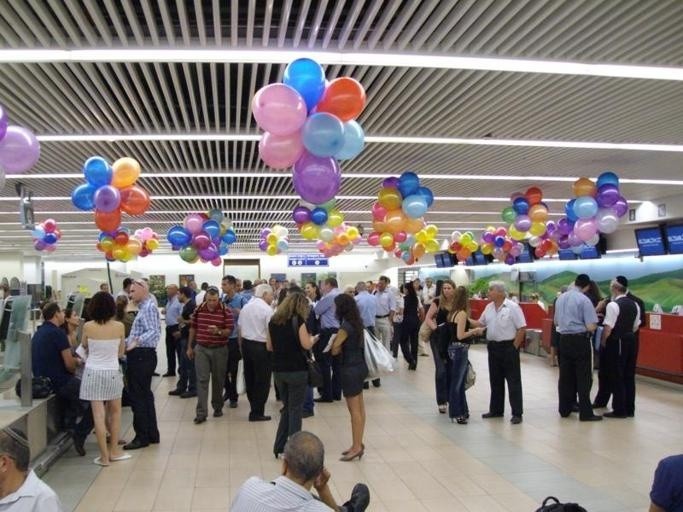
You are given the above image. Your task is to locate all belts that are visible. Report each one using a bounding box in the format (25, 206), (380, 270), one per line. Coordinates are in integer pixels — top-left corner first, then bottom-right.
(199, 344), (225, 350)
(134, 348), (155, 354)
(375, 314), (390, 318)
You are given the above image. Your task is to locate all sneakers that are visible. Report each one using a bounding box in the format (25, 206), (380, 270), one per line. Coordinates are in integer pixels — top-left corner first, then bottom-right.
(343, 483), (370, 511)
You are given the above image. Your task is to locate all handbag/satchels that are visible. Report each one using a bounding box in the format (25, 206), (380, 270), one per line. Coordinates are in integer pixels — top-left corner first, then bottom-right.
(291, 315), (324, 388)
(418, 297), (442, 342)
(393, 310), (404, 323)
(361, 328), (398, 384)
(16, 375), (54, 399)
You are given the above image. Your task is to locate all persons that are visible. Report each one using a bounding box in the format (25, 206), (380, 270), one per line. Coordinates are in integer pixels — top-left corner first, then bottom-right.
(0, 273), (527, 466)
(1, 427), (62, 510)
(227, 431), (370, 511)
(649, 454), (683, 512)
(548, 274), (647, 420)
(529, 292), (547, 311)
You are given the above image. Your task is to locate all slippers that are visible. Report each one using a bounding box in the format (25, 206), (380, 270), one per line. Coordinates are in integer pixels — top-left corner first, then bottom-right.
(94, 452), (133, 465)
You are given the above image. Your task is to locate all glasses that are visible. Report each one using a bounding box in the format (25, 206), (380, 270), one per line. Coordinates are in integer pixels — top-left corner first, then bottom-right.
(206, 287), (219, 292)
(278, 453), (286, 463)
(131, 281), (143, 287)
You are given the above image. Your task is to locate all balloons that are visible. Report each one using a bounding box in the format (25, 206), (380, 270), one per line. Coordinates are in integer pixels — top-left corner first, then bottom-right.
(259, 224), (289, 255)
(167, 209), (235, 267)
(0, 103), (40, 191)
(251, 58), (365, 204)
(33, 154), (160, 263)
(292, 197), (361, 258)
(358, 170), (627, 264)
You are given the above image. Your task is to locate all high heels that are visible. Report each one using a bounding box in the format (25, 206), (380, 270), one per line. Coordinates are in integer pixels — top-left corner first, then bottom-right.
(342, 443), (365, 455)
(438, 404), (470, 424)
(339, 450), (364, 462)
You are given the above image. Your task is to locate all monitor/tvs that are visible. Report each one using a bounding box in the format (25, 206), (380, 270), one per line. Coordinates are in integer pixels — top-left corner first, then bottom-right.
(518, 242), (533, 262)
(664, 219), (683, 254)
(579, 233), (606, 259)
(465, 255), (475, 265)
(634, 226), (667, 255)
(442, 253), (454, 266)
(434, 254), (444, 268)
(558, 250), (577, 260)
(475, 251), (488, 265)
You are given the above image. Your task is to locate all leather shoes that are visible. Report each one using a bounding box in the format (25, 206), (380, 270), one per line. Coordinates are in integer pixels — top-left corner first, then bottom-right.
(194, 394), (238, 424)
(249, 411), (271, 421)
(124, 439), (149, 449)
(164, 387), (196, 398)
(512, 415), (522, 424)
(302, 396), (342, 417)
(71, 433), (86, 456)
(106, 436), (126, 445)
(573, 401), (629, 420)
(482, 412), (504, 418)
